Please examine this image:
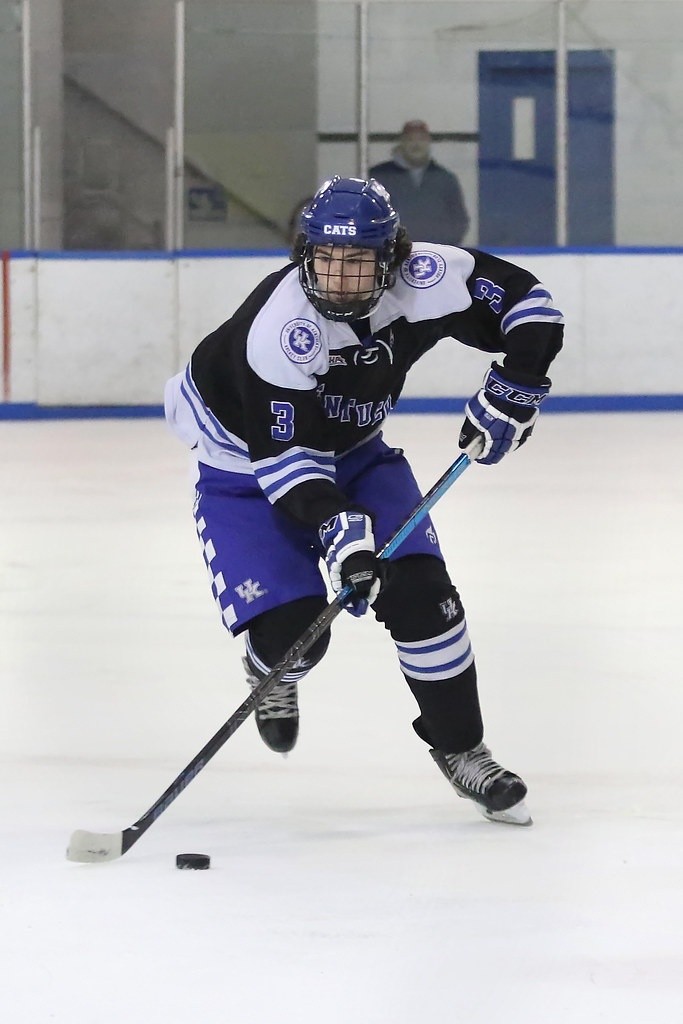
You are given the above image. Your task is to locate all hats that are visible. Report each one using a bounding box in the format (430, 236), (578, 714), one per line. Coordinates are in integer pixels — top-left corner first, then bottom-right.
(402, 120), (428, 133)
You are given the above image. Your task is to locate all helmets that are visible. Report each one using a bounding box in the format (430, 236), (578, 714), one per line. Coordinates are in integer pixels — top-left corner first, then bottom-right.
(291, 174), (413, 322)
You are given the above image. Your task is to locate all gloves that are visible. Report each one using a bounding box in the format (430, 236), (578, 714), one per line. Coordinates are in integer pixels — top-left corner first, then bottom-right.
(458, 361), (551, 465)
(318, 511), (380, 618)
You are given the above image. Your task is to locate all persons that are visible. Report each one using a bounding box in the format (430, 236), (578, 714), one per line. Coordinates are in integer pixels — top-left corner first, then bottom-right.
(362, 118), (474, 246)
(164, 175), (566, 825)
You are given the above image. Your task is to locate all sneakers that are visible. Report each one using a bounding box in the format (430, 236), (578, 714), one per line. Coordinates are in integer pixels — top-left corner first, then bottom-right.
(242, 655), (300, 753)
(412, 715), (533, 826)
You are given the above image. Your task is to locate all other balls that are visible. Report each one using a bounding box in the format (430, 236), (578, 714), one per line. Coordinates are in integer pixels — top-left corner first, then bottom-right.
(175, 853), (211, 870)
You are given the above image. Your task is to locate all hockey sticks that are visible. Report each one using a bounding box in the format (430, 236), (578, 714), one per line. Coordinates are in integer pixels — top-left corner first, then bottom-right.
(62, 432), (484, 863)
